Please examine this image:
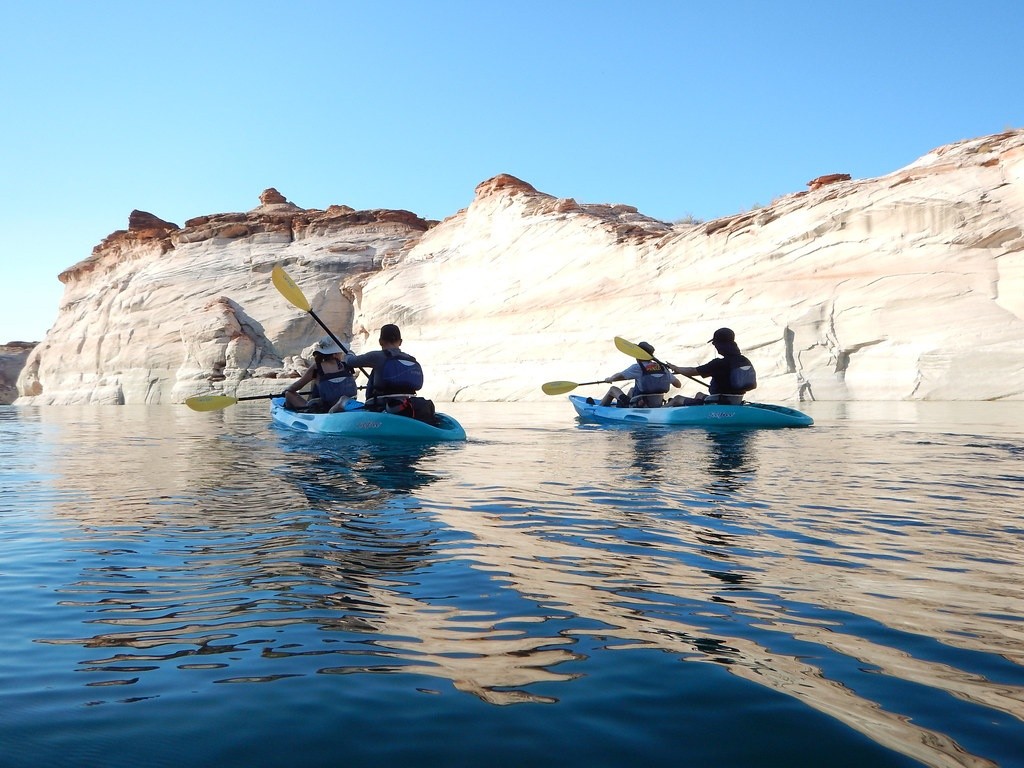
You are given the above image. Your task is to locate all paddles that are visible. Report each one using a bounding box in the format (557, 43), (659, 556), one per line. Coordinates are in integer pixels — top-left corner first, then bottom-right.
(271, 264), (370, 378)
(613, 336), (710, 387)
(185, 385), (368, 412)
(542, 372), (680, 395)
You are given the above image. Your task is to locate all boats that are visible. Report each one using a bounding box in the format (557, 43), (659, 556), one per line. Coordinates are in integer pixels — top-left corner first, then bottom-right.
(569, 393), (814, 429)
(270, 395), (467, 442)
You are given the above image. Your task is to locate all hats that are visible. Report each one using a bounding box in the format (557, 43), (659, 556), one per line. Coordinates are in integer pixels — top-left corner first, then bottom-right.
(707, 328), (734, 343)
(638, 342), (655, 355)
(312, 335), (350, 356)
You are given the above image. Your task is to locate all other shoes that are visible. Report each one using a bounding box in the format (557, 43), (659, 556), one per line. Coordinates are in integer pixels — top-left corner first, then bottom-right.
(668, 398), (672, 402)
(586, 397), (596, 405)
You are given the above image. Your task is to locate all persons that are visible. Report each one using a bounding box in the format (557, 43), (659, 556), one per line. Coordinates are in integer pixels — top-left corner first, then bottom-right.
(283, 335), (360, 413)
(328, 323), (424, 415)
(663, 327), (757, 406)
(586, 341), (681, 409)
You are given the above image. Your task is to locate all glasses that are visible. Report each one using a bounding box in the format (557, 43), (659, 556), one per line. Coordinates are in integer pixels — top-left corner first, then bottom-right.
(712, 342), (715, 345)
